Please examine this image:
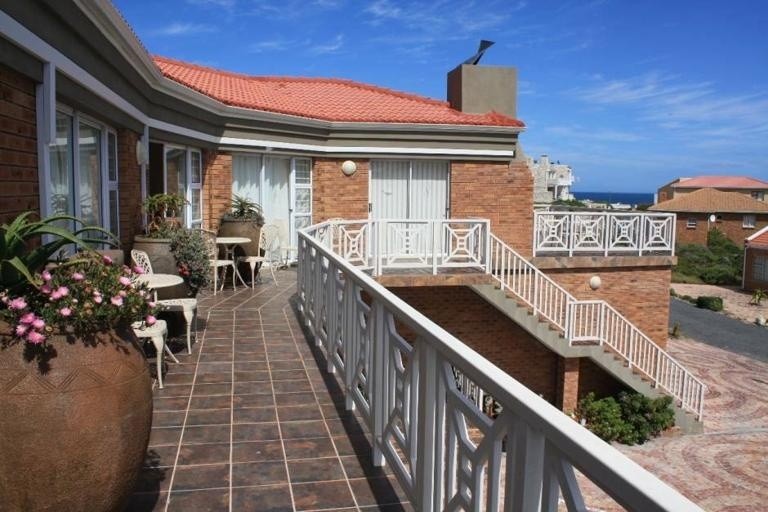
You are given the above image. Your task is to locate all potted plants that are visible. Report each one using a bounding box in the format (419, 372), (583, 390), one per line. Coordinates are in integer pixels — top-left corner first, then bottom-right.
(214, 191), (266, 283)
(129, 192), (191, 330)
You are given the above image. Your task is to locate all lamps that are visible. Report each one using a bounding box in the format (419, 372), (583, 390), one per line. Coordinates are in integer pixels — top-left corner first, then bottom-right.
(341, 161), (357, 176)
(590, 276), (601, 289)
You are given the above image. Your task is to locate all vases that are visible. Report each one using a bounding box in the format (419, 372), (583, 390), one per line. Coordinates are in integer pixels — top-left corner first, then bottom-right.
(0, 320), (153, 511)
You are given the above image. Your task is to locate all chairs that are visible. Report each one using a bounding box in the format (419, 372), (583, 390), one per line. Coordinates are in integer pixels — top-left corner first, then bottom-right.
(194, 224), (280, 296)
(130, 248), (198, 389)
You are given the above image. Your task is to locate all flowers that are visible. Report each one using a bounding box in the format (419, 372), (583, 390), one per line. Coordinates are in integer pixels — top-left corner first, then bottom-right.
(168, 227), (211, 299)
(0, 210), (162, 347)
